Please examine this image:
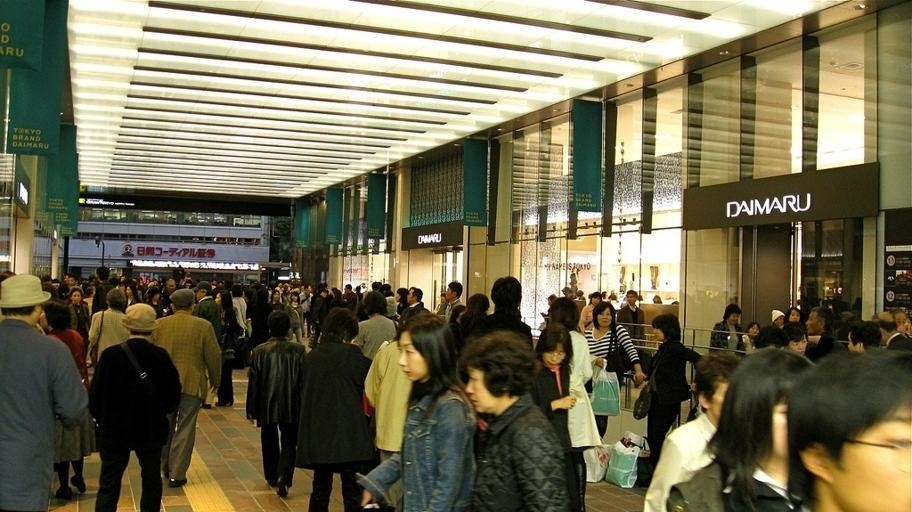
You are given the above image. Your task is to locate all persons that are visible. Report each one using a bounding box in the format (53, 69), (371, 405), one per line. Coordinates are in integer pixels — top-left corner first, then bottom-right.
(265, 281), (313, 302)
(88, 289), (129, 369)
(643, 352), (740, 511)
(547, 287), (680, 307)
(327, 284), (395, 315)
(148, 288), (222, 489)
(285, 293), (303, 344)
(616, 290), (646, 349)
(88, 303), (182, 512)
(0, 274), (89, 512)
(445, 282), (464, 333)
(432, 293), (448, 317)
(396, 286), (409, 314)
(39, 301), (87, 499)
(771, 309), (785, 328)
(463, 330), (570, 511)
(41, 266), (202, 318)
(398, 287), (429, 320)
(67, 287), (87, 349)
(353, 312), (476, 512)
(244, 310), (309, 500)
(544, 298), (610, 511)
(295, 308), (374, 512)
(805, 306), (836, 363)
(583, 301), (644, 439)
(307, 289), (329, 349)
(666, 347), (817, 512)
(349, 291), (401, 360)
(466, 276), (534, 351)
(845, 324), (870, 355)
(191, 281), (223, 409)
(215, 290), (241, 408)
(232, 285), (248, 366)
(786, 306), (804, 322)
(246, 288), (273, 347)
(781, 321), (809, 357)
(872, 312), (912, 350)
(362, 306), (430, 509)
(647, 312), (703, 473)
(531, 323), (576, 418)
(458, 292), (490, 328)
(709, 303), (745, 353)
(579, 292), (603, 332)
(447, 318), (466, 351)
(787, 349), (912, 511)
(753, 324), (791, 350)
(744, 323), (761, 349)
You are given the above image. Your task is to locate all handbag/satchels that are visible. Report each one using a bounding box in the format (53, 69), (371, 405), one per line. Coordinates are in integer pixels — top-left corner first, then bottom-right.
(606, 441), (640, 489)
(90, 311), (104, 368)
(583, 447), (608, 482)
(633, 343), (666, 420)
(589, 360), (620, 416)
(606, 323), (632, 375)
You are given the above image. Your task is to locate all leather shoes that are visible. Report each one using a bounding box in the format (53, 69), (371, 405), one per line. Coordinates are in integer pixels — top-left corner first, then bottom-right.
(215, 400), (233, 407)
(278, 477), (288, 496)
(71, 475), (86, 492)
(55, 486), (71, 499)
(169, 478), (186, 486)
(268, 478), (277, 487)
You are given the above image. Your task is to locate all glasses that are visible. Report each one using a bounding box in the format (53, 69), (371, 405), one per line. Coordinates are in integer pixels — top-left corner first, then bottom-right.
(845, 435), (911, 471)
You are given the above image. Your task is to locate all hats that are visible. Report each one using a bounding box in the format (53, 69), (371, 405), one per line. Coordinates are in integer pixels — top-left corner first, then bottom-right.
(120, 304), (159, 331)
(0, 274), (51, 308)
(170, 289), (194, 306)
(196, 281), (211, 290)
(771, 310), (784, 322)
